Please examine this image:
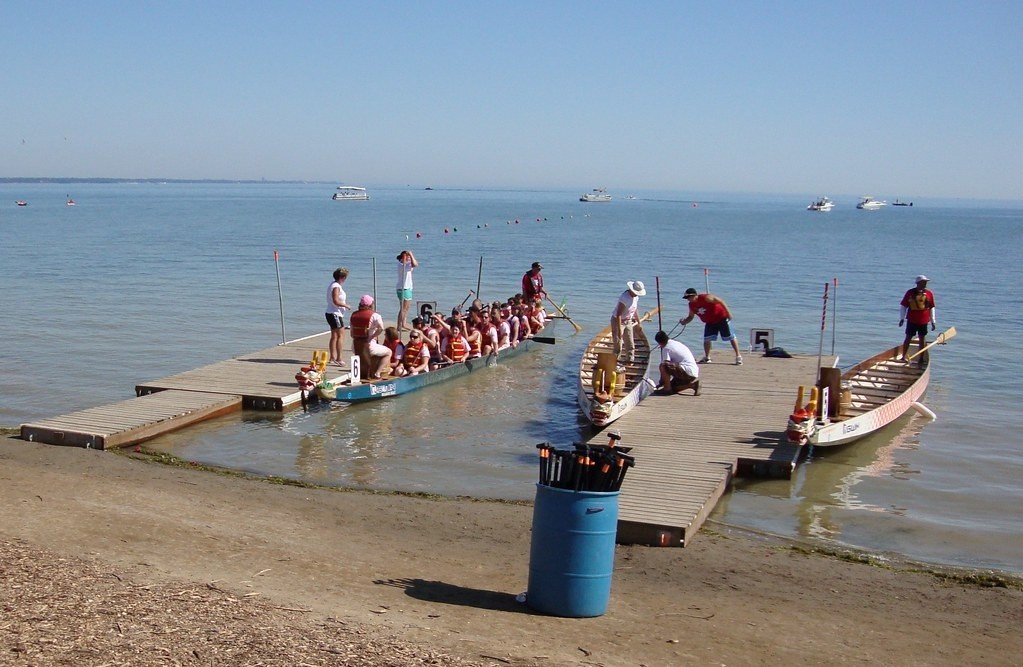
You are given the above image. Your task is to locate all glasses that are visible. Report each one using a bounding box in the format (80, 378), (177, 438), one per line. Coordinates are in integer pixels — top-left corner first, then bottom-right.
(410, 335), (418, 339)
(481, 316), (488, 319)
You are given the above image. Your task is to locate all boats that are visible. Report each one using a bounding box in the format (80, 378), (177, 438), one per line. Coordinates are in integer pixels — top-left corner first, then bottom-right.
(892, 202), (913, 206)
(66, 199), (75, 206)
(856, 201), (880, 210)
(808, 336), (930, 447)
(577, 317), (651, 425)
(807, 197), (836, 209)
(579, 188), (613, 202)
(18, 201), (27, 206)
(817, 207), (831, 212)
(625, 195), (636, 200)
(873, 201), (887, 206)
(332, 186), (370, 201)
(315, 295), (566, 404)
(425, 187), (434, 191)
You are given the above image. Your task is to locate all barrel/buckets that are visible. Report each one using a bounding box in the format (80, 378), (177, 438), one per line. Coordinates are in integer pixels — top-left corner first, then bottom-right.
(527, 483), (621, 618)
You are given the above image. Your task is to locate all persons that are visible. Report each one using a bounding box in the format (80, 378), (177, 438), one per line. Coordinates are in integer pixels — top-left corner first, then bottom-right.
(325, 268), (351, 366)
(898, 275), (936, 364)
(655, 331), (702, 396)
(350, 295), (392, 378)
(396, 251), (419, 331)
(679, 288), (743, 365)
(610, 280), (646, 366)
(383, 264), (549, 379)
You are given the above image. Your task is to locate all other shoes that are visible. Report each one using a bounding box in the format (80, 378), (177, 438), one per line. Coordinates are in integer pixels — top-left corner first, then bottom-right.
(654, 388), (674, 394)
(398, 327), (412, 331)
(329, 359), (346, 367)
(694, 380), (702, 396)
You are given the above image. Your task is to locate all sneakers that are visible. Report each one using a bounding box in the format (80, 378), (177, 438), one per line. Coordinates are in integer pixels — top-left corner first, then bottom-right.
(699, 358), (712, 363)
(736, 356), (743, 365)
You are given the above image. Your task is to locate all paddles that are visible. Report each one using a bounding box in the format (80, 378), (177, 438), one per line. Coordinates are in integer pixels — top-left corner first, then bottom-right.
(480, 305), (489, 310)
(437, 355), (478, 365)
(534, 433), (637, 492)
(906, 326), (957, 364)
(519, 336), (556, 345)
(632, 305), (663, 328)
(462, 289), (476, 305)
(545, 292), (582, 332)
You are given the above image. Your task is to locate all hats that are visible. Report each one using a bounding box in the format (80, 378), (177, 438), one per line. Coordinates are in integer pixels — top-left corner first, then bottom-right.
(627, 281), (646, 296)
(532, 262), (544, 269)
(915, 275), (930, 283)
(360, 295), (374, 306)
(682, 288), (697, 299)
(452, 307), (460, 313)
